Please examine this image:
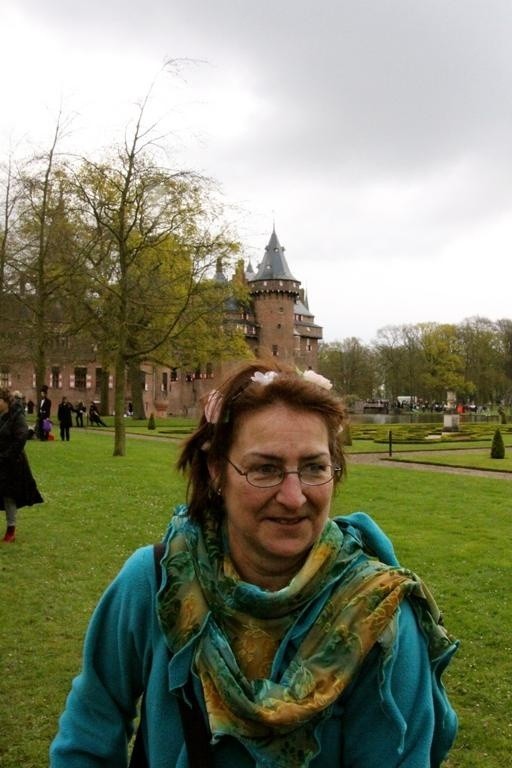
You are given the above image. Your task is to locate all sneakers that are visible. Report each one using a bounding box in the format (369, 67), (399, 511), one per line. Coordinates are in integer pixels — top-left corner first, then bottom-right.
(4, 526), (16, 544)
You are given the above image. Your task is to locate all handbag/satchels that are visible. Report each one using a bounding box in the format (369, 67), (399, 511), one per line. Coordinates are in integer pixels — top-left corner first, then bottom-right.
(43, 419), (51, 431)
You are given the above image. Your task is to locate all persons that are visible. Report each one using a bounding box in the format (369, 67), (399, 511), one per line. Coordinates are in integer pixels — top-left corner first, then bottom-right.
(50, 348), (460, 768)
(365, 397), (490, 415)
(0, 383), (107, 543)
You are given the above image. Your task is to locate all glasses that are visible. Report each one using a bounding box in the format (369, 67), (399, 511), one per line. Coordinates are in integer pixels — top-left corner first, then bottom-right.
(223, 452), (343, 489)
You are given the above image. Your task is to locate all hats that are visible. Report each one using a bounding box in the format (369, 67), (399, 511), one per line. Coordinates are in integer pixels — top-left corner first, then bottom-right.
(40, 385), (48, 394)
